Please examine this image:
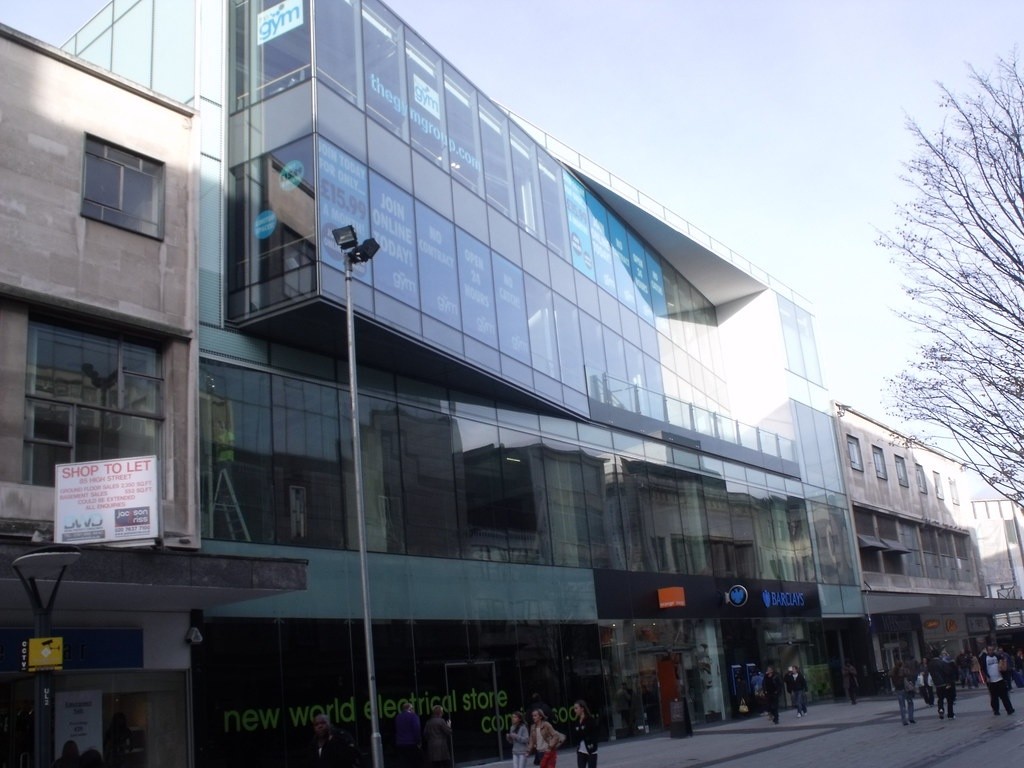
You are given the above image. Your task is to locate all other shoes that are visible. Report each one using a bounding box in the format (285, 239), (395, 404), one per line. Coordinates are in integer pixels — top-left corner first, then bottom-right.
(948, 715), (956, 719)
(904, 723), (909, 725)
(852, 701), (857, 705)
(1007, 709), (1015, 715)
(797, 713), (807, 718)
(994, 711), (1001, 715)
(939, 709), (944, 719)
(910, 720), (916, 723)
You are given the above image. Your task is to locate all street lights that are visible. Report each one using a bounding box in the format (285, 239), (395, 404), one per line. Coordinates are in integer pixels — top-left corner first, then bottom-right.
(333, 225), (382, 768)
(82, 363), (126, 461)
(10, 544), (82, 768)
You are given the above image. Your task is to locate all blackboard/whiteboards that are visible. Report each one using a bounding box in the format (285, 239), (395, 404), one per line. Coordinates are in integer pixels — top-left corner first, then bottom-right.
(670, 697), (686, 723)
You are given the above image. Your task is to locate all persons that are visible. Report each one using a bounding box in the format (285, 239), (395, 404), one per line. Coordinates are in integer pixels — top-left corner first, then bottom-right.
(526, 708), (558, 768)
(395, 703), (422, 768)
(505, 711), (530, 768)
(308, 713), (363, 768)
(425, 705), (452, 767)
(610, 642), (1024, 737)
(0, 703), (134, 768)
(570, 700), (598, 767)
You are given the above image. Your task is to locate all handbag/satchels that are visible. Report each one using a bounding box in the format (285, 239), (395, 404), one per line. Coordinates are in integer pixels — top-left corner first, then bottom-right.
(554, 732), (566, 748)
(916, 675), (924, 687)
(739, 698), (748, 712)
(999, 660), (1008, 671)
(904, 677), (915, 692)
(928, 673), (935, 687)
